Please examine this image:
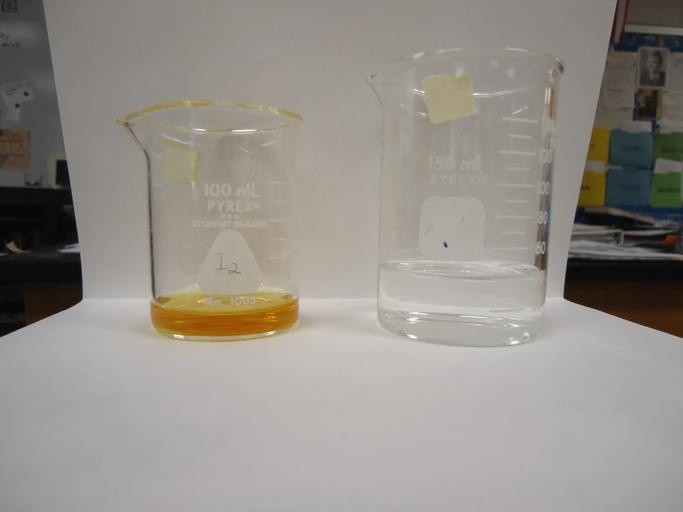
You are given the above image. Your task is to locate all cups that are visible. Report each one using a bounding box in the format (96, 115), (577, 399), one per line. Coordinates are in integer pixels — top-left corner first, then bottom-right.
(113, 99), (306, 343)
(365, 45), (564, 348)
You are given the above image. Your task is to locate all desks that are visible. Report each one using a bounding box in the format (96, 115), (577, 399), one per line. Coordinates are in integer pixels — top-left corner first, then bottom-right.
(565, 258), (683, 337)
(0, 247), (80, 326)
